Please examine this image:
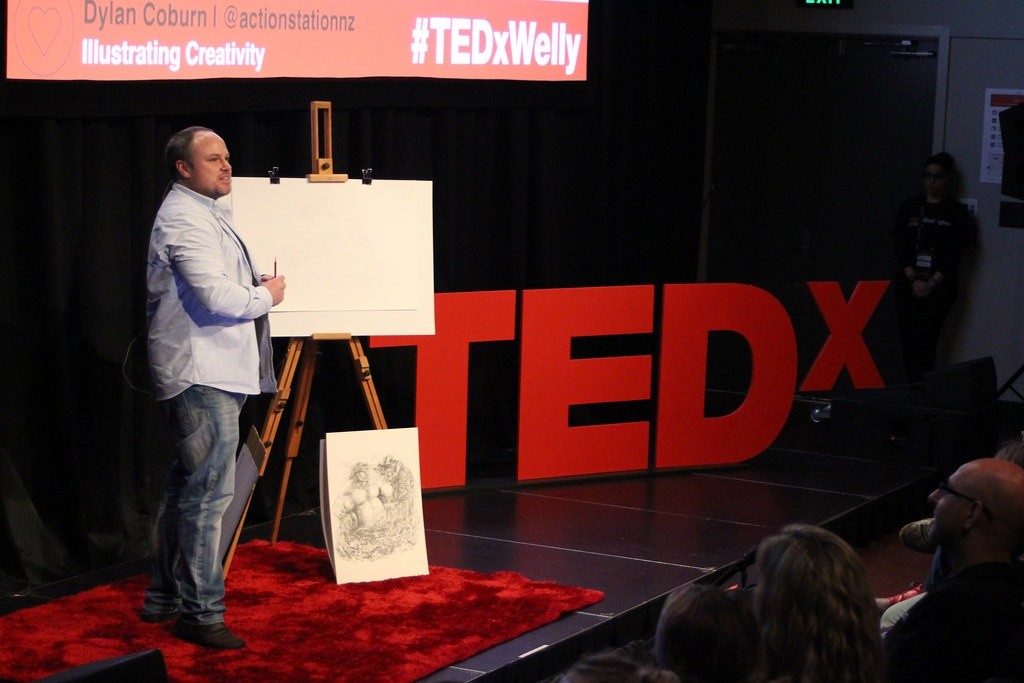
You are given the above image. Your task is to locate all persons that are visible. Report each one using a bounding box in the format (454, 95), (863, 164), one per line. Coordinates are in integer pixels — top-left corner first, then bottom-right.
(892, 151), (978, 400)
(140, 126), (286, 648)
(553, 438), (1024, 683)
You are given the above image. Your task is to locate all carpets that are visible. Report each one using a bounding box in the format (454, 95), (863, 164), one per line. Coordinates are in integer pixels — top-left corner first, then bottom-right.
(0, 536), (605, 683)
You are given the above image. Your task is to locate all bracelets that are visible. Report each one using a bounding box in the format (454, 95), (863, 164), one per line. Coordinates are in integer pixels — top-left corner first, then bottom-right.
(907, 272), (919, 284)
(931, 276), (939, 286)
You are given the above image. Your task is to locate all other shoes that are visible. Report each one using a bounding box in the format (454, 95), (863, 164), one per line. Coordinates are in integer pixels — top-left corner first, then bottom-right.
(899, 518), (937, 553)
(175, 619), (245, 649)
(141, 612), (178, 622)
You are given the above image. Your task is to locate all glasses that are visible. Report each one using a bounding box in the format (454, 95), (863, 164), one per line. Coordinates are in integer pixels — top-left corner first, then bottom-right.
(939, 479), (993, 521)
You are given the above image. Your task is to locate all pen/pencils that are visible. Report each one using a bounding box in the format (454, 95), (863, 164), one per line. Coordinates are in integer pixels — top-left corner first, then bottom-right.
(273, 256), (278, 279)
